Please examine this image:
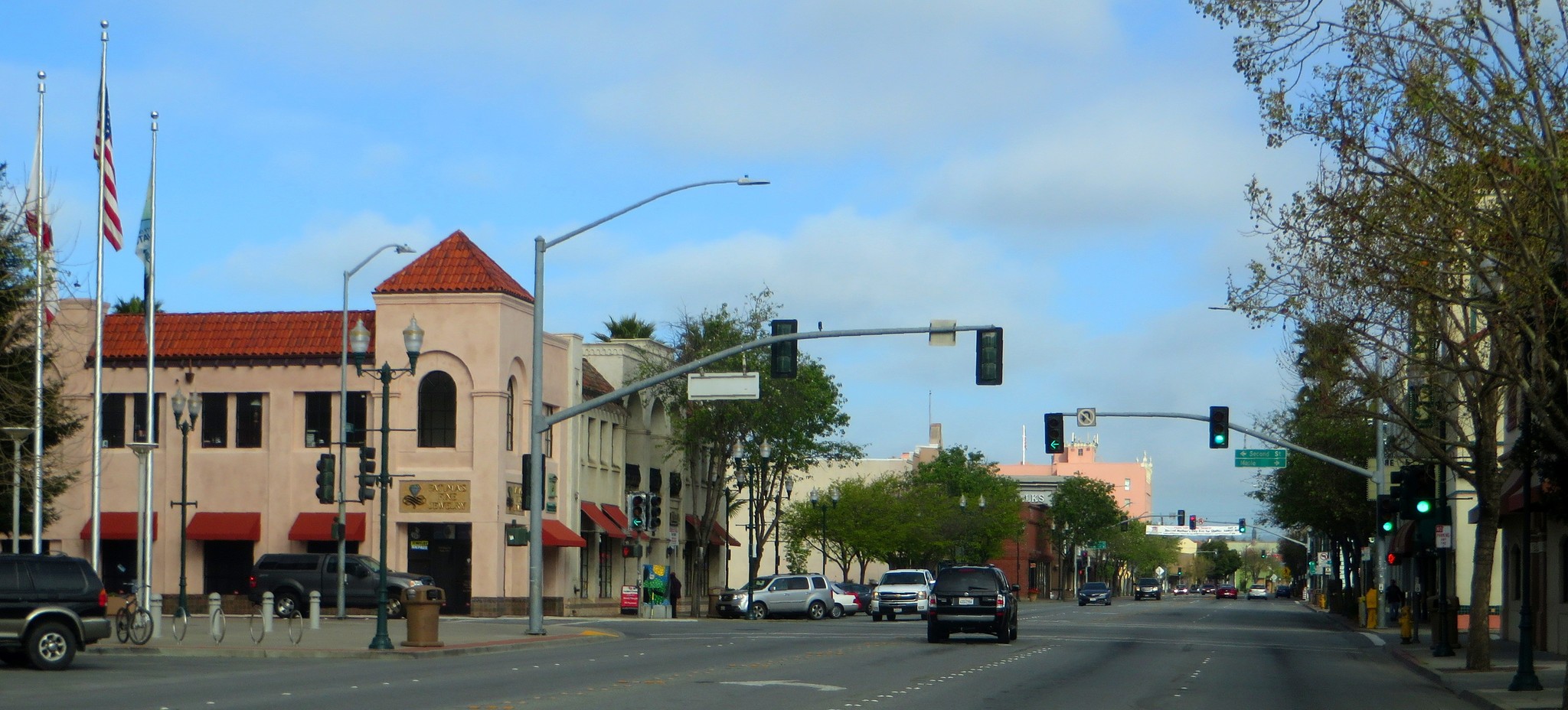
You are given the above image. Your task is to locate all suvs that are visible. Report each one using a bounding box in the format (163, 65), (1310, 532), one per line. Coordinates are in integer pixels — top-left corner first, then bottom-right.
(0, 548), (113, 672)
(715, 559), (1021, 645)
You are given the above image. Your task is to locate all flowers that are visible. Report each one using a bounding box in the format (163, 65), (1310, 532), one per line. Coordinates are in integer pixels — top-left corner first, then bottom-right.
(1029, 587), (1039, 594)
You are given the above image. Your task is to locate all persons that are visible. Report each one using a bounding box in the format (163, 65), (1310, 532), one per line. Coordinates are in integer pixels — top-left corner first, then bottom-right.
(1385, 579), (1403, 620)
(670, 572), (682, 618)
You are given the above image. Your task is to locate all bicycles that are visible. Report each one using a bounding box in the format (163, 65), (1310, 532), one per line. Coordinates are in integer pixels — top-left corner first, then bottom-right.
(115, 582), (155, 646)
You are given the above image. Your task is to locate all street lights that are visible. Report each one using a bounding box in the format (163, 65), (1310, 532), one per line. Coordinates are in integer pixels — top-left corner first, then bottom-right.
(334, 241), (417, 618)
(171, 386), (201, 618)
(524, 173), (772, 637)
(731, 437), (841, 621)
(347, 310), (427, 651)
(1477, 253), (1543, 688)
(1404, 256), (1457, 657)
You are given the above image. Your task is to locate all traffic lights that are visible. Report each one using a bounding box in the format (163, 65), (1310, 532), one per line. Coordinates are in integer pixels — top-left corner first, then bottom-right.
(622, 494), (662, 558)
(506, 528), (528, 546)
(1376, 462), (1437, 565)
(1209, 406), (1229, 449)
(1044, 413), (1064, 454)
(314, 454), (335, 504)
(1080, 550), (1088, 567)
(359, 446), (376, 504)
(1189, 515), (1246, 533)
(1080, 570), (1085, 576)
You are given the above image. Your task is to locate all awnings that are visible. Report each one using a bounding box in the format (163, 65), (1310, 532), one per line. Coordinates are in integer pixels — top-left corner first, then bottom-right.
(701, 516), (741, 546)
(186, 512), (260, 541)
(581, 500), (627, 538)
(685, 514), (725, 545)
(542, 519), (586, 547)
(288, 512), (365, 542)
(1470, 457), (1562, 524)
(1386, 520), (1415, 556)
(80, 512), (157, 541)
(601, 503), (650, 540)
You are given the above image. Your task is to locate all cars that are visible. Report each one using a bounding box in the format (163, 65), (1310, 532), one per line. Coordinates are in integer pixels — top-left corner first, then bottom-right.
(1076, 578), (1292, 608)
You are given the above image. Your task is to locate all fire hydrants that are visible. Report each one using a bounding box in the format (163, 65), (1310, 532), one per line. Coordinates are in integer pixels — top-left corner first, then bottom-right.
(1397, 605), (1414, 645)
(1318, 593), (1326, 610)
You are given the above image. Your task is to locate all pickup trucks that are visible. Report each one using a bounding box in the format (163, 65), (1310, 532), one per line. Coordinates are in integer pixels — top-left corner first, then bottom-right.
(248, 552), (437, 620)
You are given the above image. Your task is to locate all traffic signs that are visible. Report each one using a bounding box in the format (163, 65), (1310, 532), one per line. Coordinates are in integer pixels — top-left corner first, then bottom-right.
(1234, 449), (1287, 468)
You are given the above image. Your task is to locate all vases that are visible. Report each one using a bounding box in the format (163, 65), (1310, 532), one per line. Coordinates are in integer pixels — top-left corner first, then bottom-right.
(1030, 594), (1037, 601)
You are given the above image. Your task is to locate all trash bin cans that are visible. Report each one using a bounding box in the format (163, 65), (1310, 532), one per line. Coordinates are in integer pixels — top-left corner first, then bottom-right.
(1358, 597), (1368, 628)
(1327, 579), (1343, 613)
(401, 584), (445, 648)
(1426, 595), (1462, 649)
